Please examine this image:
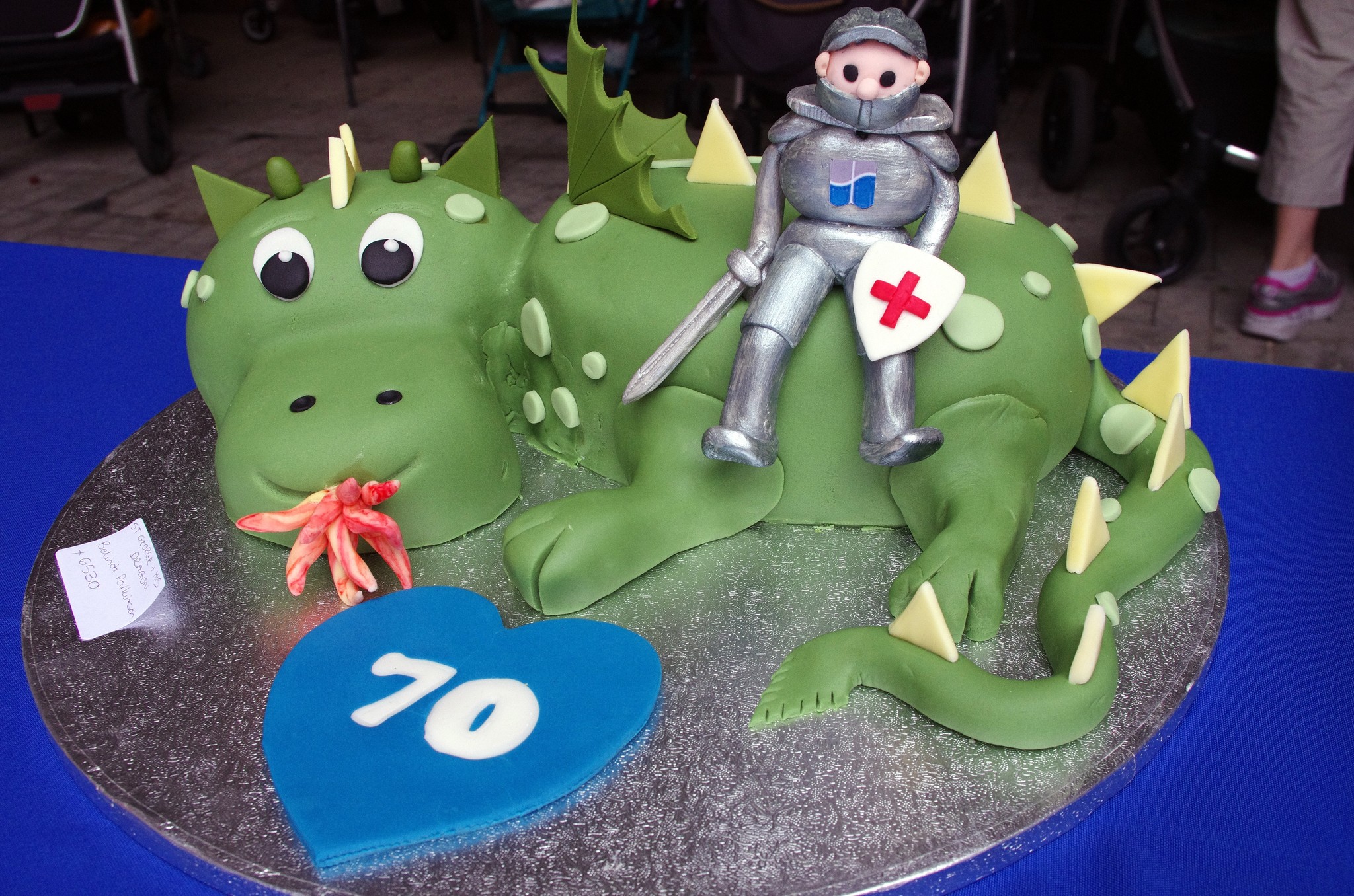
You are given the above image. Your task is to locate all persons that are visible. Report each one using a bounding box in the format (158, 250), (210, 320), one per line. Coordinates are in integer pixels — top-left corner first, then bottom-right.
(700, 6), (961, 468)
(1242, 0), (1354, 339)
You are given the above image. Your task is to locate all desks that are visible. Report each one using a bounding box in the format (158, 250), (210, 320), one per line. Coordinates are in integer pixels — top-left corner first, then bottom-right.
(0, 242), (1353, 896)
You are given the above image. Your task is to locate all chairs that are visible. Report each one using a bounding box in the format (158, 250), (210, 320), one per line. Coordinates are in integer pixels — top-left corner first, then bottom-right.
(478, 0), (647, 125)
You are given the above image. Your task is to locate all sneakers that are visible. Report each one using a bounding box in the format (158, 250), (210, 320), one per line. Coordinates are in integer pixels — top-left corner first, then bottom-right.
(1241, 252), (1343, 341)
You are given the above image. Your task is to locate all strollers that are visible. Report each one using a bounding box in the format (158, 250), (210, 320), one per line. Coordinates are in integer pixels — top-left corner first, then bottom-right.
(0, 1), (178, 176)
(439, 0), (1265, 285)
